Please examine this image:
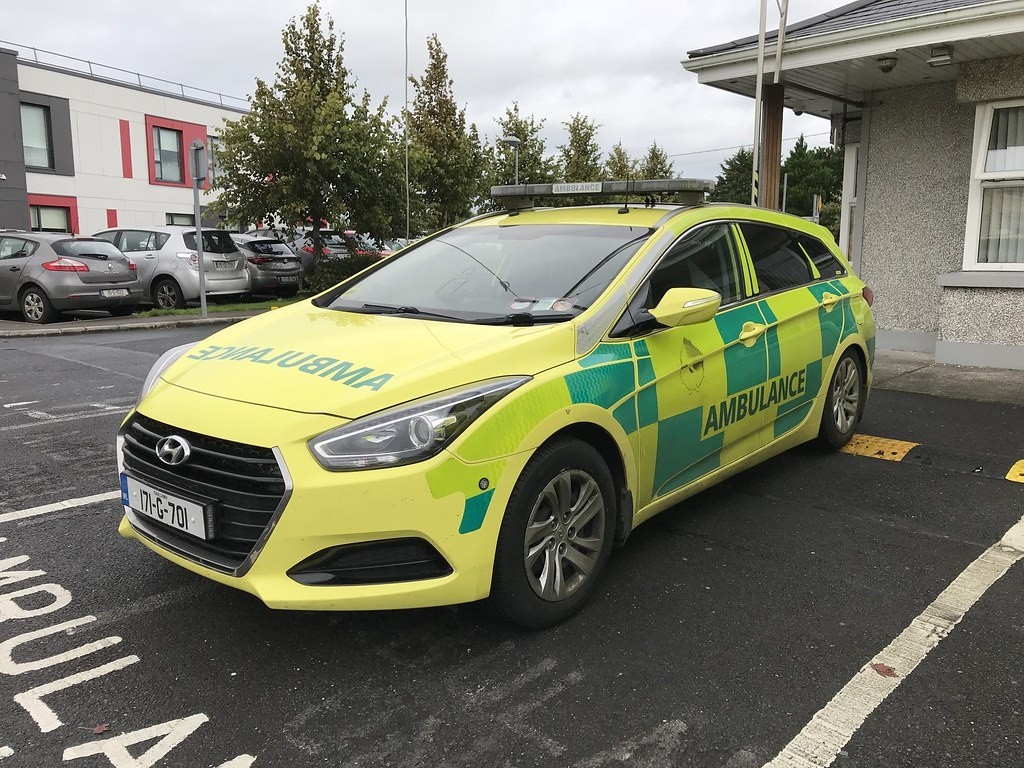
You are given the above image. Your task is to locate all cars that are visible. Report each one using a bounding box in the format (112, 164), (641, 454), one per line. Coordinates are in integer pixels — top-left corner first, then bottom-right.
(243, 227), (422, 278)
(0, 229), (139, 324)
(92, 226), (253, 309)
(229, 234), (301, 299)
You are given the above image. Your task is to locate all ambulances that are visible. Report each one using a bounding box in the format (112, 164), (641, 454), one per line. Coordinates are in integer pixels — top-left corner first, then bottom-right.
(116, 180), (877, 630)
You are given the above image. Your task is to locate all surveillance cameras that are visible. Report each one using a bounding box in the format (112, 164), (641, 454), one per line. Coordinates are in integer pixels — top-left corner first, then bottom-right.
(793, 106), (804, 116)
(878, 59), (897, 73)
(1, 175), (6, 180)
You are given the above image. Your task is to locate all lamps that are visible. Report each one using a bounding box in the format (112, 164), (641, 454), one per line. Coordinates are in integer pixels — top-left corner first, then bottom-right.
(927, 47), (955, 67)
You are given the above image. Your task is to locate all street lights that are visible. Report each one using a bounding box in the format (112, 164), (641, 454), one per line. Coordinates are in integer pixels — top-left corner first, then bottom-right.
(501, 133), (521, 186)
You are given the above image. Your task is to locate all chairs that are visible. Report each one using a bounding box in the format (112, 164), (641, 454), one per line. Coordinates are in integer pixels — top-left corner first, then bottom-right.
(123, 235), (141, 251)
(3, 244), (28, 259)
